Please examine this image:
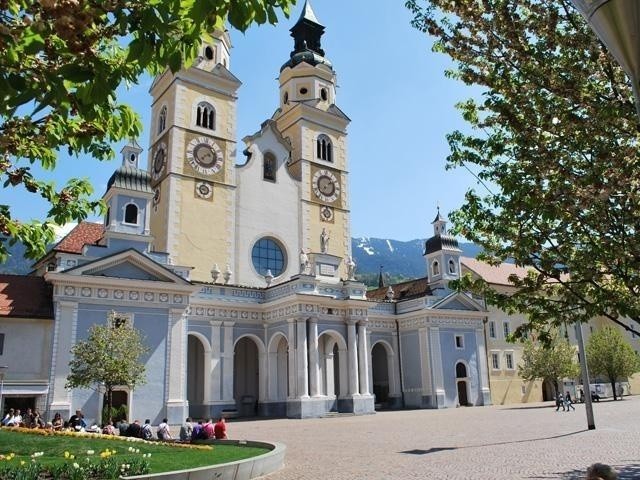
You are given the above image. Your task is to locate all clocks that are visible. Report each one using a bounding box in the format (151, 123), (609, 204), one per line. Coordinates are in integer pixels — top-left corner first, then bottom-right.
(193, 178), (214, 202)
(150, 143), (166, 181)
(187, 136), (224, 176)
(152, 184), (161, 206)
(313, 170), (342, 203)
(319, 205), (335, 222)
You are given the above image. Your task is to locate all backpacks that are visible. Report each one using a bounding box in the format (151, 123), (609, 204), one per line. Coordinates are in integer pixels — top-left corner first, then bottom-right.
(157, 430), (163, 438)
(198, 429), (208, 440)
(103, 426), (149, 439)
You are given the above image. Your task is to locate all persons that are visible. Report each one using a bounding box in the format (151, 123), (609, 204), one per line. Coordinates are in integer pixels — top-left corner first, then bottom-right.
(555, 391), (565, 411)
(618, 384), (625, 401)
(564, 391), (576, 412)
(318, 226), (333, 254)
(0, 406), (228, 441)
(345, 255), (355, 280)
(299, 248), (309, 274)
(593, 392), (601, 402)
(579, 388), (585, 403)
